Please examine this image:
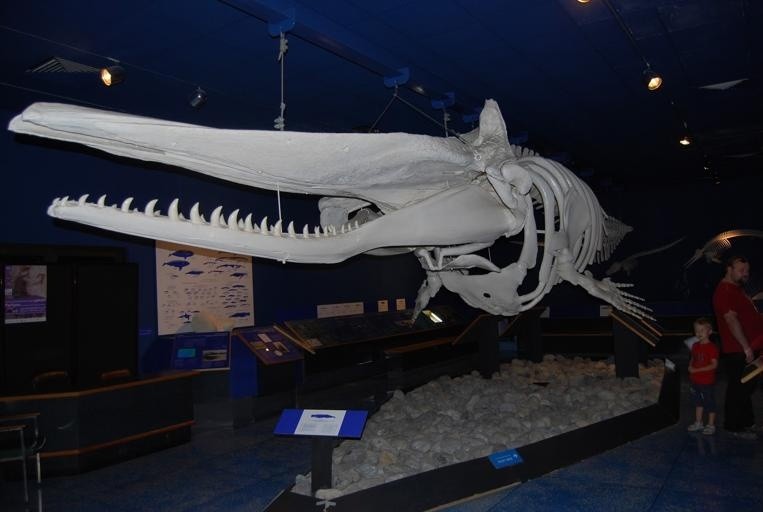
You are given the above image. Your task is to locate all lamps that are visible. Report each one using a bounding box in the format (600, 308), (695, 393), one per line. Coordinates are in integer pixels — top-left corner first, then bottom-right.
(99, 61), (207, 111)
(642, 66), (693, 148)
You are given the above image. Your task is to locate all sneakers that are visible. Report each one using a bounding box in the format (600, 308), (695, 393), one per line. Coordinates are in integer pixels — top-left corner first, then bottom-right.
(724, 424), (763, 441)
(703, 424), (716, 435)
(688, 422), (704, 431)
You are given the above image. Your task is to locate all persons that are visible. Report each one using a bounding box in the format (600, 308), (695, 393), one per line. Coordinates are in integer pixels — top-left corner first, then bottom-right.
(687, 257), (763, 440)
